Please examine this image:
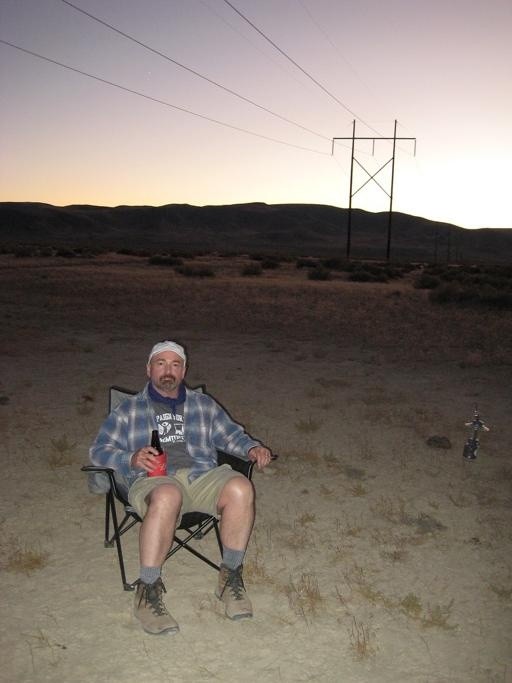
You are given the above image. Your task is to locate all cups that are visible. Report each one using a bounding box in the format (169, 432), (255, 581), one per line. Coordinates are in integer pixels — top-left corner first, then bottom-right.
(145, 452), (169, 477)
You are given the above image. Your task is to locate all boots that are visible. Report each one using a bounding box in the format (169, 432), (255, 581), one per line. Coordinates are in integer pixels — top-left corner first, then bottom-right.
(130, 576), (180, 637)
(215, 564), (253, 620)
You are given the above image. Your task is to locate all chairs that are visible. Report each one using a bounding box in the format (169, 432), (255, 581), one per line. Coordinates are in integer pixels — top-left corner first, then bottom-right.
(73, 383), (280, 595)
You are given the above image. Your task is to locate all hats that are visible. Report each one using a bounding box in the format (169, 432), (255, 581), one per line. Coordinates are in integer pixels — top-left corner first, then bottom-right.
(148, 341), (187, 368)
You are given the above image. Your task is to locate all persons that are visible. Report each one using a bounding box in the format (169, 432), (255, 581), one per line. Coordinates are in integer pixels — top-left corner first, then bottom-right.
(90, 340), (273, 637)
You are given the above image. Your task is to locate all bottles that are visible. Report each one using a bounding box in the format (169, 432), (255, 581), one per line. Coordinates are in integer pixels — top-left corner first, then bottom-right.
(147, 430), (162, 455)
(462, 437), (481, 459)
